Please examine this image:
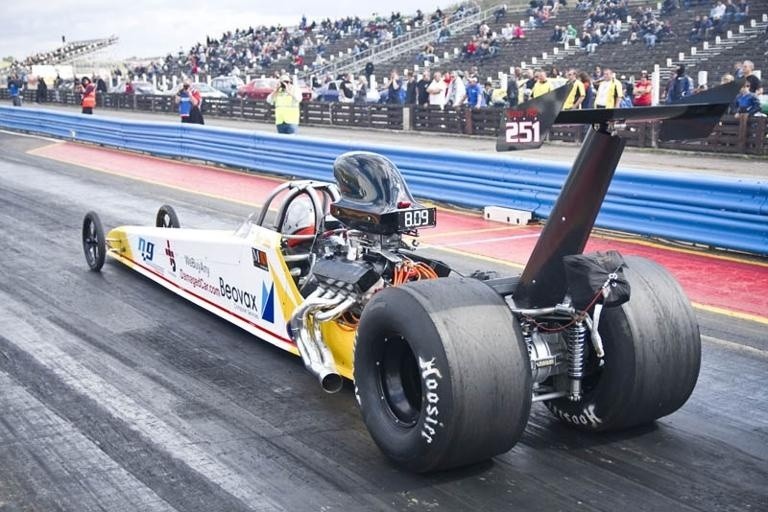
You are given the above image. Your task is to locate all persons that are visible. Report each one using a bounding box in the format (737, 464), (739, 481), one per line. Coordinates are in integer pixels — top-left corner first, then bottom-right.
(290, 7), (465, 65)
(309, 71), (368, 117)
(663, 60), (767, 116)
(175, 81), (202, 124)
(173, 26), (290, 98)
(459, 5), (524, 61)
(7, 49), (63, 106)
(278, 200), (325, 254)
(73, 54), (175, 115)
(528, 0), (750, 54)
(385, 68), (654, 116)
(267, 74), (303, 135)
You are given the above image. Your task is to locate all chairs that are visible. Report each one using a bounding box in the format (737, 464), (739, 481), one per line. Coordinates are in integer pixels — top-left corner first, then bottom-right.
(150, 0), (767, 116)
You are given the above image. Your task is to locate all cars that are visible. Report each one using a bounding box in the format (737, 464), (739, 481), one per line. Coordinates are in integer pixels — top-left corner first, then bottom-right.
(113, 81), (163, 95)
(313, 80), (381, 103)
(238, 79), (312, 101)
(171, 82), (227, 98)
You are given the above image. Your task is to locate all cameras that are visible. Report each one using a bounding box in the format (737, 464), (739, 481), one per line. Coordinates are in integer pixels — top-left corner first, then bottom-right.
(280, 81), (286, 89)
(184, 84), (190, 90)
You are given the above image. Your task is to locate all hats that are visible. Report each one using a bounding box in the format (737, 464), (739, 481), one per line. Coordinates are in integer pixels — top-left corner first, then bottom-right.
(279, 75), (290, 83)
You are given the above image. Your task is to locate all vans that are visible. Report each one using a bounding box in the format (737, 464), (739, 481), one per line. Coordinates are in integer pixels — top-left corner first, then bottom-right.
(211, 76), (245, 97)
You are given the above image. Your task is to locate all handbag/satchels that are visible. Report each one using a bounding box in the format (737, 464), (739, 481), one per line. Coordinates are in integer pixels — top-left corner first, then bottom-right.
(190, 106), (204, 124)
